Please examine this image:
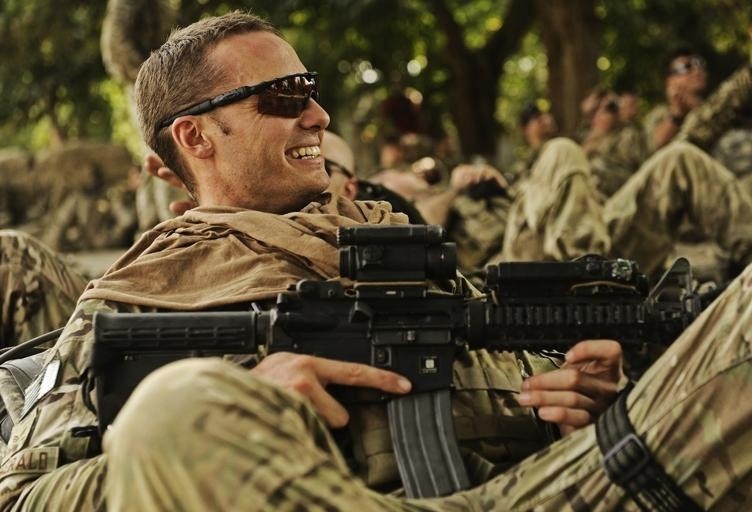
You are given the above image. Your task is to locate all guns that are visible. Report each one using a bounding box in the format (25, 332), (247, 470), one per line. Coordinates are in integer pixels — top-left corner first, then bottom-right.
(94, 224), (730, 500)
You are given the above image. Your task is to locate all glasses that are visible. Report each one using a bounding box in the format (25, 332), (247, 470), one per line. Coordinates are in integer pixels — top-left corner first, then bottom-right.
(161, 70), (320, 127)
